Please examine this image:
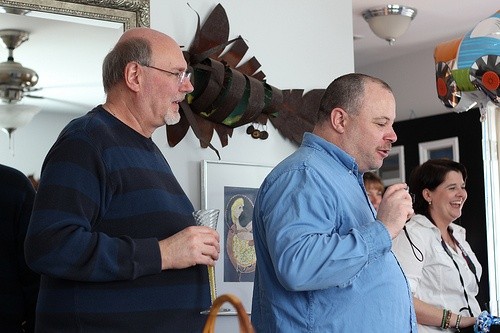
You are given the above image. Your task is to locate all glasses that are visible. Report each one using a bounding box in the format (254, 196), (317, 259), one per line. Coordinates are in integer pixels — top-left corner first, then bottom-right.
(145, 64), (192, 84)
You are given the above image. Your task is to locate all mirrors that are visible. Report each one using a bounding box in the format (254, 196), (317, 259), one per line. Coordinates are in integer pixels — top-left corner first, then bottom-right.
(0, 0), (150, 333)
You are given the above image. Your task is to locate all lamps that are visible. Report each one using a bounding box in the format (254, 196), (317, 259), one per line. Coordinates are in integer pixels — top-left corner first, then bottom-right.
(0, 29), (40, 142)
(361, 5), (417, 47)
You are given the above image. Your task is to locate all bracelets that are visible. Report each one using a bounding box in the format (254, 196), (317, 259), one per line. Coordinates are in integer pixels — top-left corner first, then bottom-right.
(456, 314), (462, 332)
(442, 309), (446, 328)
(445, 310), (452, 328)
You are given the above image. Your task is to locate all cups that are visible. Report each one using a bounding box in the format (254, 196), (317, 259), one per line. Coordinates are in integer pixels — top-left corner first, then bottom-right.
(192, 209), (220, 231)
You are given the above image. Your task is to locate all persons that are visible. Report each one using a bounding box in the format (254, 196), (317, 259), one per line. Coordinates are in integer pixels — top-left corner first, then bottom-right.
(0, 164), (37, 333)
(250, 73), (419, 333)
(24, 27), (219, 333)
(391, 158), (482, 333)
(363, 172), (384, 215)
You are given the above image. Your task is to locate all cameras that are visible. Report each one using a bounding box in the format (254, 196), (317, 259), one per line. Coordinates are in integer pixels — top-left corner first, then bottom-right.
(383, 184), (411, 225)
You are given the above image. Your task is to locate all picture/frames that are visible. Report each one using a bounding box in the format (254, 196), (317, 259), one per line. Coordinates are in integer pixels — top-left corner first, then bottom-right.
(199, 159), (276, 317)
